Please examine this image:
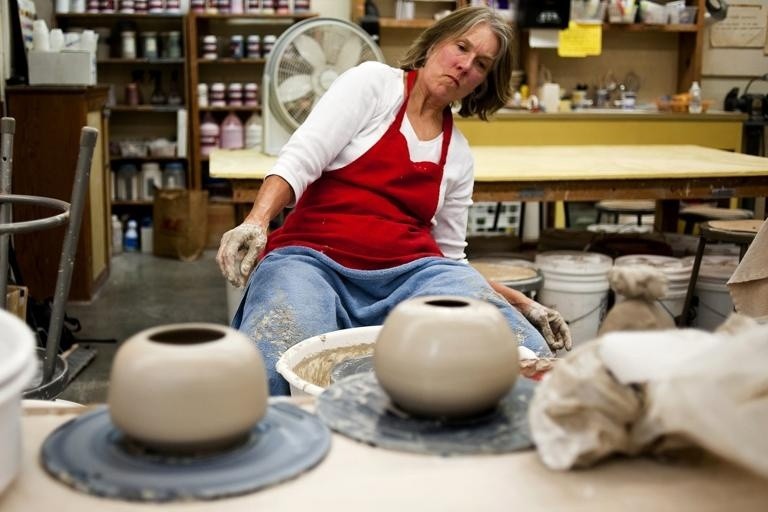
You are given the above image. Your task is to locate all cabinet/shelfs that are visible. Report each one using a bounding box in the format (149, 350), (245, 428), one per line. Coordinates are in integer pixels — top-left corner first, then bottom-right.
(50, 0), (193, 254)
(188, 2), (356, 207)
(353, 0), (703, 111)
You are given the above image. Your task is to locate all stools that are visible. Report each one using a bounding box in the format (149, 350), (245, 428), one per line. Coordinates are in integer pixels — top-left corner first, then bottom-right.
(594, 201), (657, 229)
(679, 217), (764, 326)
(677, 204), (754, 246)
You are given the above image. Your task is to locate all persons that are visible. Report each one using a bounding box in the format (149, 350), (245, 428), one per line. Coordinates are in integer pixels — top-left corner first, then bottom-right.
(215, 5), (573, 397)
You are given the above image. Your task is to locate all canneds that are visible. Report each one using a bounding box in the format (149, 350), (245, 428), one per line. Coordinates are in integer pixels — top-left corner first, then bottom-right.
(121, 29), (136, 58)
(161, 30), (180, 59)
(125, 83), (142, 105)
(139, 30), (157, 58)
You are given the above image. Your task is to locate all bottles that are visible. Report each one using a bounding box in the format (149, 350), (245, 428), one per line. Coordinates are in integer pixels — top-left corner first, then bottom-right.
(140, 217), (154, 253)
(688, 81), (702, 114)
(168, 70), (183, 104)
(150, 71), (166, 104)
(219, 114), (243, 150)
(244, 112), (262, 151)
(124, 218), (138, 251)
(110, 214), (122, 253)
(116, 164), (139, 201)
(164, 162), (185, 189)
(198, 113), (220, 155)
(138, 163), (161, 200)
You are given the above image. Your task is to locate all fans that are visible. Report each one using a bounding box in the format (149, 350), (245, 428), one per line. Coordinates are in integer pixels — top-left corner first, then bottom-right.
(258, 16), (385, 156)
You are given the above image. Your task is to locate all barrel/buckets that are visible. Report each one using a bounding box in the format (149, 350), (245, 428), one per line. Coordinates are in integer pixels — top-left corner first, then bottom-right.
(613, 252), (685, 327)
(686, 256), (738, 334)
(535, 250), (612, 357)
(273, 323), (384, 394)
(471, 257), (544, 305)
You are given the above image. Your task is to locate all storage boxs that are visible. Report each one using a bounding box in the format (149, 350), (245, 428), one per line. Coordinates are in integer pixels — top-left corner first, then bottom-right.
(149, 189), (208, 259)
